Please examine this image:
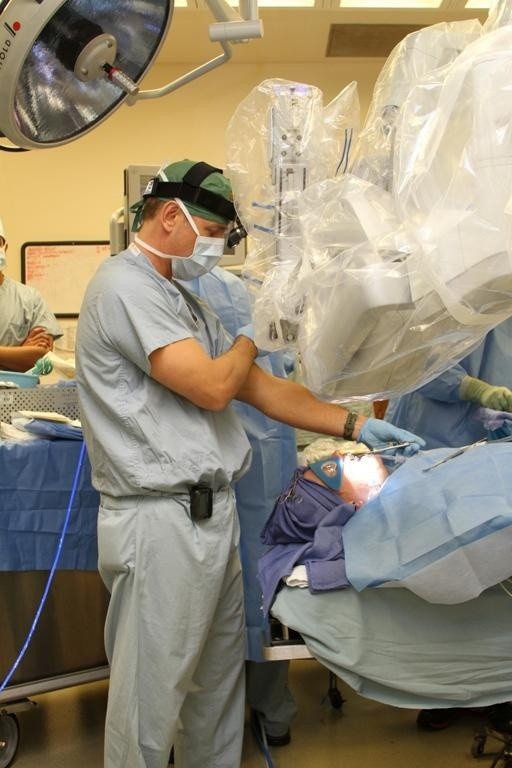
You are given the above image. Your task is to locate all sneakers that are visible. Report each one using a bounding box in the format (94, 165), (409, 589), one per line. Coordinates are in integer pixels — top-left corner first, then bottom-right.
(416, 709), (460, 731)
(266, 727), (290, 747)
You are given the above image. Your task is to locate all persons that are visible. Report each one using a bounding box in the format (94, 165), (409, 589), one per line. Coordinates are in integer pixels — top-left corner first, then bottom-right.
(71, 156), (428, 768)
(0, 230), (64, 428)
(378, 313), (511, 455)
(182, 261), (294, 746)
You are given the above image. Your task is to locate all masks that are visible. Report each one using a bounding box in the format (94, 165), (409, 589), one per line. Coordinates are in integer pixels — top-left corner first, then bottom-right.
(0, 244), (8, 271)
(164, 200), (225, 281)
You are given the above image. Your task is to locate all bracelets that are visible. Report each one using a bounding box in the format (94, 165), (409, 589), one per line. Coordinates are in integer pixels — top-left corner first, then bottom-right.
(342, 411), (365, 443)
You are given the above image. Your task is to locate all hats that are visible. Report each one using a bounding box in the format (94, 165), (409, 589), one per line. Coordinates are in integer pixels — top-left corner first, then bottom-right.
(154, 160), (233, 225)
(0, 221), (6, 243)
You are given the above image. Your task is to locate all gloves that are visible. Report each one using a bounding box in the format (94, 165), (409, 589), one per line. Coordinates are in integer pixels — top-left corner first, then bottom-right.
(458, 375), (512, 412)
(235, 323), (271, 357)
(355, 417), (426, 467)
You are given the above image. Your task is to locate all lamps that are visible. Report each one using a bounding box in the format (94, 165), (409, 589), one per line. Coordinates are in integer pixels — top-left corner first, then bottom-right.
(0, 1), (174, 152)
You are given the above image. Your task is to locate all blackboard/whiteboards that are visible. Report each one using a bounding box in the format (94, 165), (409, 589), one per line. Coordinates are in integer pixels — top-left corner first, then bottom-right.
(21, 240), (111, 318)
